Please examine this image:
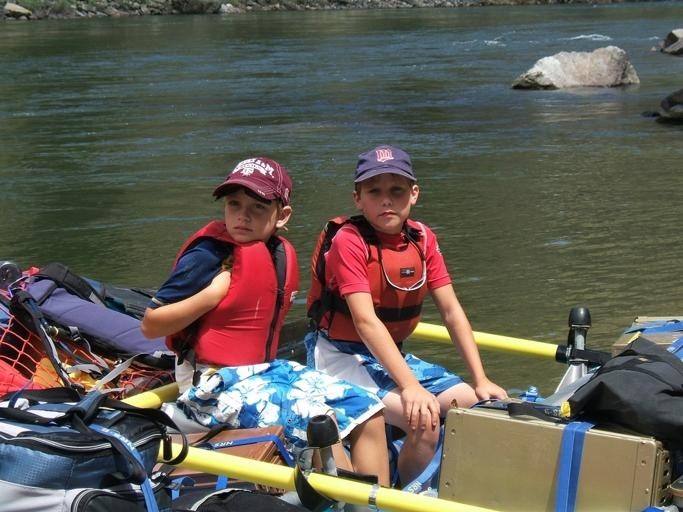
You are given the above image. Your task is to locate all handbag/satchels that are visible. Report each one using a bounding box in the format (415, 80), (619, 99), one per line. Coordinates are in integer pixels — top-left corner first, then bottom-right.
(566, 334), (683, 481)
(0, 381), (180, 512)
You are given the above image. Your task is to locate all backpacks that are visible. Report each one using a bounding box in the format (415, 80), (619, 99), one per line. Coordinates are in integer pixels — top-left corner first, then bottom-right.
(9, 262), (177, 371)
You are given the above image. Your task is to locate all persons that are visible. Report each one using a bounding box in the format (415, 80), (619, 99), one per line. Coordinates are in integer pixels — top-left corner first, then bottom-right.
(300, 141), (512, 497)
(139, 153), (392, 489)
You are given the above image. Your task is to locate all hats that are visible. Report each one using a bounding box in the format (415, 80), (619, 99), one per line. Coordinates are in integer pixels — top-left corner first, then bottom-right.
(352, 143), (418, 186)
(212, 154), (294, 207)
